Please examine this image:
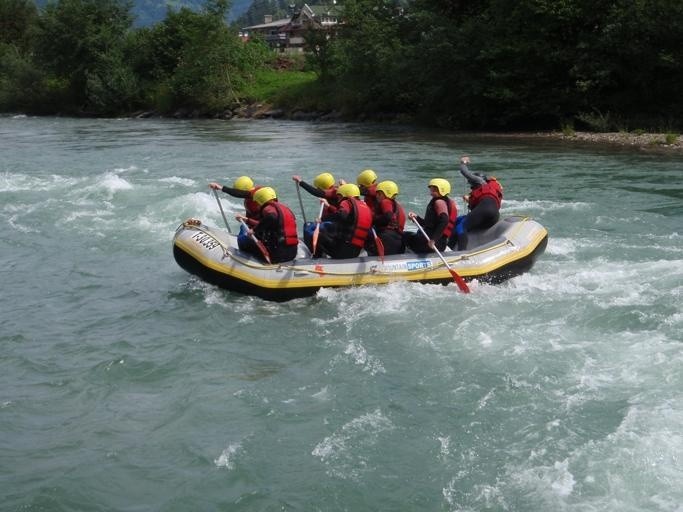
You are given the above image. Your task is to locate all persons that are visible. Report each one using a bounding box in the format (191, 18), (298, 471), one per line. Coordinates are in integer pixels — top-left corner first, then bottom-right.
(207, 176), (262, 238)
(235, 186), (298, 265)
(403, 178), (458, 259)
(447, 156), (503, 252)
(292, 169), (406, 260)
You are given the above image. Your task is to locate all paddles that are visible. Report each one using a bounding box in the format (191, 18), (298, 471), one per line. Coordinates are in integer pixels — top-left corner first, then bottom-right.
(340, 177), (385, 265)
(235, 216), (271, 265)
(412, 216), (471, 295)
(312, 200), (324, 255)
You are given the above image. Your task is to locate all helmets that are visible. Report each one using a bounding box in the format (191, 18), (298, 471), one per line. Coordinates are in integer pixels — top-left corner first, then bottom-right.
(428, 178), (451, 197)
(234, 175), (254, 191)
(468, 172), (487, 184)
(376, 180), (399, 199)
(358, 169), (377, 186)
(253, 185), (277, 204)
(336, 183), (360, 198)
(314, 172), (336, 191)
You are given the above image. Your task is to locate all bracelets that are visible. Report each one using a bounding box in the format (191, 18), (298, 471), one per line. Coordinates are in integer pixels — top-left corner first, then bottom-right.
(461, 161), (468, 164)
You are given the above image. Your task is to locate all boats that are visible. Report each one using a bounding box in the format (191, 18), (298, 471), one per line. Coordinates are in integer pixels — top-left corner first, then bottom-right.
(171, 216), (550, 303)
